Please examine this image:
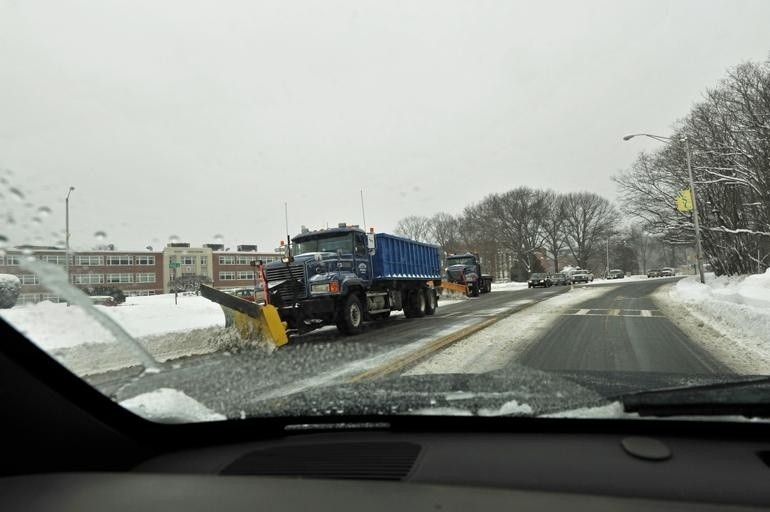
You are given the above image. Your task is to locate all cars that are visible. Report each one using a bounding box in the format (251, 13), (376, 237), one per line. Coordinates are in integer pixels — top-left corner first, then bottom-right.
(528, 266), (676, 289)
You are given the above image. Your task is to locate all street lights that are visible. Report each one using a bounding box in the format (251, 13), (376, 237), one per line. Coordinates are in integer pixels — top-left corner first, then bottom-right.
(65, 186), (76, 307)
(623, 133), (706, 284)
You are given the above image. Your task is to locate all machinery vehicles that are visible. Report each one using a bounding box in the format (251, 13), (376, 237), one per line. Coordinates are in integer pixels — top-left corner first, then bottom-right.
(200, 222), (442, 347)
(426, 250), (496, 297)
